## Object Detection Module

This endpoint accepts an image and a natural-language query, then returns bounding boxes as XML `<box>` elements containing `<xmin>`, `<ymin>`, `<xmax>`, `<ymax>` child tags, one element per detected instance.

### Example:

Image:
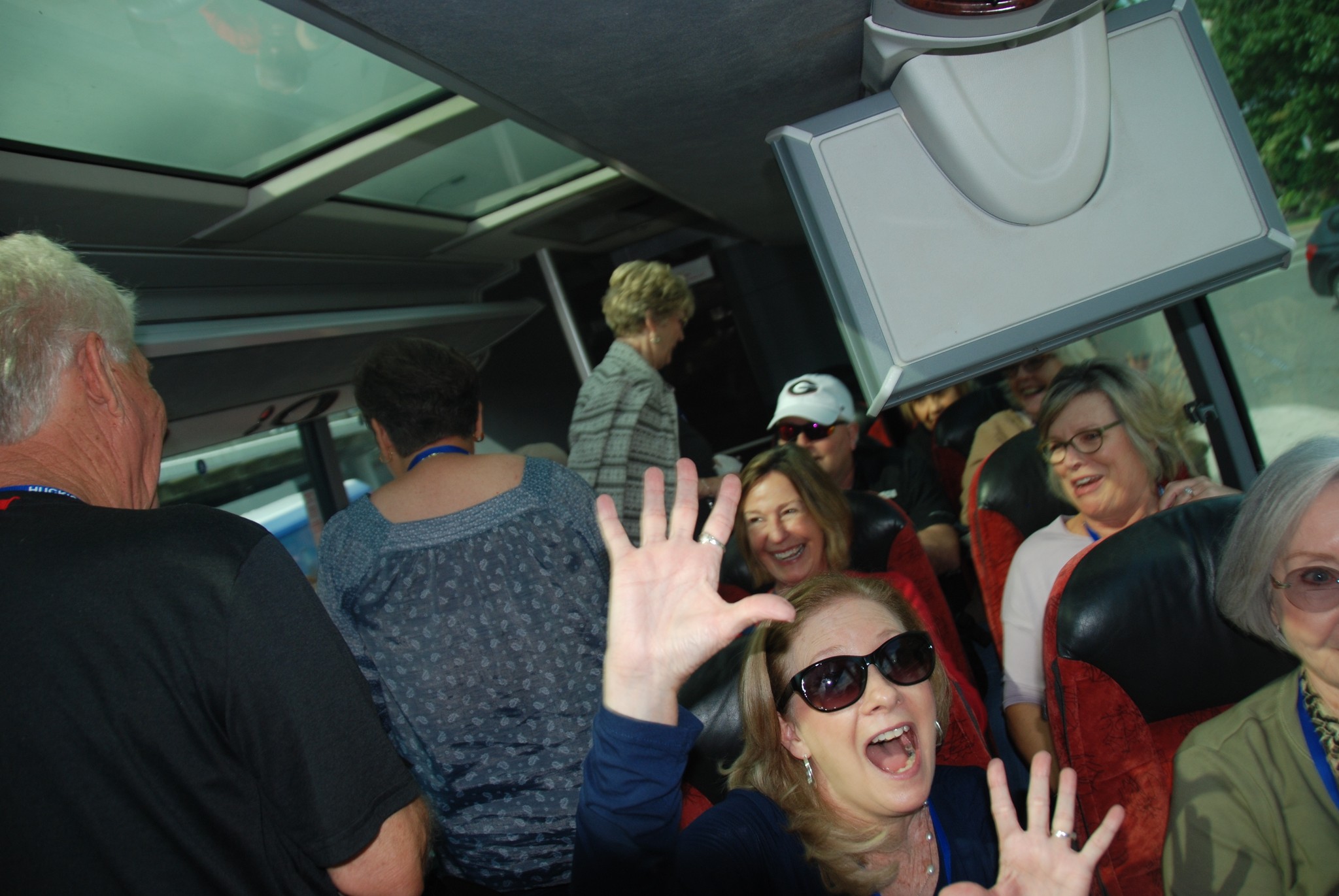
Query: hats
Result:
<box><xmin>767</xmin><ymin>373</ymin><xmax>856</xmax><ymax>430</ymax></box>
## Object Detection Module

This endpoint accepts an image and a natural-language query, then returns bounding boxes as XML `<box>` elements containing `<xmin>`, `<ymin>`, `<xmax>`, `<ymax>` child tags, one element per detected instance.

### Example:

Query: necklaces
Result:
<box><xmin>917</xmin><ymin>799</ymin><xmax>935</xmax><ymax>896</ymax></box>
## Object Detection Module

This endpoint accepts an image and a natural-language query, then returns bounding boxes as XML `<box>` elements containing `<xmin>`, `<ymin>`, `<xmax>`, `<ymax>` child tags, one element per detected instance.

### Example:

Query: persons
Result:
<box><xmin>311</xmin><ymin>260</ymin><xmax>1339</xmax><ymax>896</ymax></box>
<box><xmin>0</xmin><ymin>228</ymin><xmax>434</xmax><ymax>895</ymax></box>
<box><xmin>572</xmin><ymin>453</ymin><xmax>1125</xmax><ymax>896</ymax></box>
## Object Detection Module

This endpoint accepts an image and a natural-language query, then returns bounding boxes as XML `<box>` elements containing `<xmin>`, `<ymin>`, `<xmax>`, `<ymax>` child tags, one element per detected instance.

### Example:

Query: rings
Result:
<box><xmin>1183</xmin><ymin>486</ymin><xmax>1195</xmax><ymax>498</ymax></box>
<box><xmin>697</xmin><ymin>529</ymin><xmax>729</xmax><ymax>555</ymax></box>
<box><xmin>1051</xmin><ymin>830</ymin><xmax>1077</xmax><ymax>840</ymax></box>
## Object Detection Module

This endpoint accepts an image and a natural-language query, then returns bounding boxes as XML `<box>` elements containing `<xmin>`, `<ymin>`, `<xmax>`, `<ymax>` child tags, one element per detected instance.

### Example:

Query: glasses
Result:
<box><xmin>1269</xmin><ymin>565</ymin><xmax>1339</xmax><ymax>611</ymax></box>
<box><xmin>780</xmin><ymin>629</ymin><xmax>935</xmax><ymax>716</ymax></box>
<box><xmin>774</xmin><ymin>420</ymin><xmax>848</xmax><ymax>440</ymax></box>
<box><xmin>1042</xmin><ymin>418</ymin><xmax>1122</xmax><ymax>464</ymax></box>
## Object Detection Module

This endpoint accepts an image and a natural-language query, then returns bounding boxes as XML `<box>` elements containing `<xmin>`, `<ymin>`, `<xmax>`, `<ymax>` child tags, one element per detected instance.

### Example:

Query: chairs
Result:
<box><xmin>679</xmin><ymin>385</ymin><xmax>1301</xmax><ymax>896</ymax></box>
